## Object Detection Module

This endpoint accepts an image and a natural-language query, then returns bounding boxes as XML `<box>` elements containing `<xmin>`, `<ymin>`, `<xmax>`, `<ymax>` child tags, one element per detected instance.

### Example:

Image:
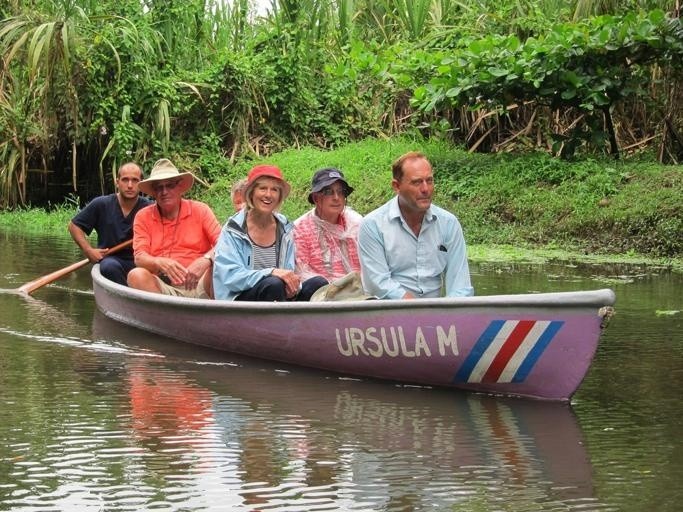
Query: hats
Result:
<box><xmin>308</xmin><ymin>167</ymin><xmax>354</xmax><ymax>205</ymax></box>
<box><xmin>244</xmin><ymin>165</ymin><xmax>291</xmax><ymax>210</ymax></box>
<box><xmin>137</xmin><ymin>158</ymin><xmax>194</xmax><ymax>198</ymax></box>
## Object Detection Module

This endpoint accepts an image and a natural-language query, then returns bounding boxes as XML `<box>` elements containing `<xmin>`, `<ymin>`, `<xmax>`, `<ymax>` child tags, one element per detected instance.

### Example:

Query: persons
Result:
<box><xmin>356</xmin><ymin>153</ymin><xmax>475</xmax><ymax>300</ymax></box>
<box><xmin>292</xmin><ymin>168</ymin><xmax>364</xmax><ymax>282</ymax></box>
<box><xmin>125</xmin><ymin>159</ymin><xmax>222</xmax><ymax>299</ymax></box>
<box><xmin>230</xmin><ymin>178</ymin><xmax>250</xmax><ymax>213</ymax></box>
<box><xmin>211</xmin><ymin>164</ymin><xmax>326</xmax><ymax>301</ymax></box>
<box><xmin>68</xmin><ymin>162</ymin><xmax>152</xmax><ymax>287</ymax></box>
<box><xmin>131</xmin><ymin>348</ymin><xmax>209</xmax><ymax>472</ymax></box>
<box><xmin>292</xmin><ymin>419</ymin><xmax>345</xmax><ymax>488</ymax></box>
<box><xmin>213</xmin><ymin>394</ymin><xmax>300</xmax><ymax>509</ymax></box>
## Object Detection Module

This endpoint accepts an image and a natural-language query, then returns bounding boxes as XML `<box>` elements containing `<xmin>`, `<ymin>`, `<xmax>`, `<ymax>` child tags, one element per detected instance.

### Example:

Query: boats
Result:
<box><xmin>90</xmin><ymin>263</ymin><xmax>615</xmax><ymax>403</ymax></box>
<box><xmin>81</xmin><ymin>308</ymin><xmax>599</xmax><ymax>490</ymax></box>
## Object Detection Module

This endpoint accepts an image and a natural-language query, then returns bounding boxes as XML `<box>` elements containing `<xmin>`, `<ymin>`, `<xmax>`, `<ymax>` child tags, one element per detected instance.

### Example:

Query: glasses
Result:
<box><xmin>320</xmin><ymin>186</ymin><xmax>350</xmax><ymax>198</ymax></box>
<box><xmin>152</xmin><ymin>181</ymin><xmax>176</xmax><ymax>191</ymax></box>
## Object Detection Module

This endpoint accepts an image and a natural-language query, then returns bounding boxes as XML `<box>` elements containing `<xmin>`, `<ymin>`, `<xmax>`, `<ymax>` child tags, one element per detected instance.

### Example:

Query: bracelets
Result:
<box><xmin>203</xmin><ymin>252</ymin><xmax>211</xmax><ymax>260</ymax></box>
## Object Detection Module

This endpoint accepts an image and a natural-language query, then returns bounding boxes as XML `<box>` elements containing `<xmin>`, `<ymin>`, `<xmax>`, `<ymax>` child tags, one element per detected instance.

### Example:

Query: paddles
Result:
<box><xmin>19</xmin><ymin>238</ymin><xmax>136</xmax><ymax>299</ymax></box>
